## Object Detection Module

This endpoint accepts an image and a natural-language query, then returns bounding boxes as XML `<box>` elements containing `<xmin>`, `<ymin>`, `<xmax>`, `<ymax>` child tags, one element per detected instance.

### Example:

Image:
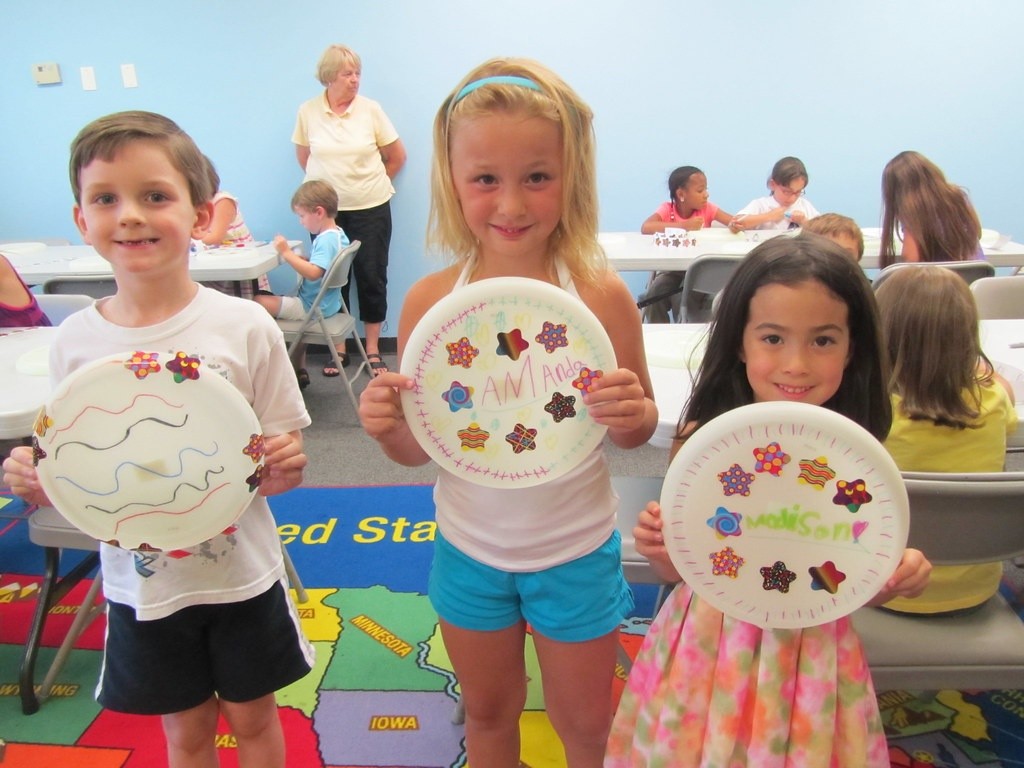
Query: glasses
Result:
<box><xmin>778</xmin><ymin>184</ymin><xmax>805</xmax><ymax>198</ymax></box>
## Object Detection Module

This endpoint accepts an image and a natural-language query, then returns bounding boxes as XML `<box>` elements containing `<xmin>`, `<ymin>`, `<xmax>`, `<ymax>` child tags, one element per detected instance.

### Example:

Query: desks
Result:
<box><xmin>0</xmin><ymin>326</ymin><xmax>103</xmax><ymax>715</ymax></box>
<box><xmin>631</xmin><ymin>318</ymin><xmax>1023</xmax><ymax>467</ymax></box>
<box><xmin>1</xmin><ymin>240</ymin><xmax>302</xmax><ymax>303</ymax></box>
<box><xmin>597</xmin><ymin>228</ymin><xmax>1023</xmax><ymax>313</ymax></box>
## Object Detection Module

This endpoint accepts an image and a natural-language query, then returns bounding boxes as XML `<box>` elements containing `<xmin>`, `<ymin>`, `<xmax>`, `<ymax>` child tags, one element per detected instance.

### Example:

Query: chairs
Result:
<box><xmin>850</xmin><ymin>470</ymin><xmax>1023</xmax><ymax>692</ymax></box>
<box><xmin>678</xmin><ymin>252</ymin><xmax>748</xmax><ymax>322</ymax></box>
<box><xmin>868</xmin><ymin>261</ymin><xmax>995</xmax><ymax>287</ymax></box>
<box><xmin>267</xmin><ymin>239</ymin><xmax>374</xmax><ymax>419</ymax></box>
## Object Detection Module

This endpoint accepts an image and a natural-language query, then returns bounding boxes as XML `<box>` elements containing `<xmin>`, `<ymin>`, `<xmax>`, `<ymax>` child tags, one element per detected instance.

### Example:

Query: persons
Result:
<box><xmin>1</xmin><ymin>111</ymin><xmax>312</xmax><ymax>768</ymax></box>
<box><xmin>868</xmin><ymin>262</ymin><xmax>1019</xmax><ymax>621</ymax></box>
<box><xmin>644</xmin><ymin>151</ymin><xmax>986</xmax><ymax>324</ymax></box>
<box><xmin>0</xmin><ymin>256</ymin><xmax>52</xmax><ymax>328</ymax></box>
<box><xmin>192</xmin><ymin>152</ymin><xmax>273</xmax><ymax>300</ymax></box>
<box><xmin>293</xmin><ymin>45</ymin><xmax>407</xmax><ymax>375</ymax></box>
<box><xmin>255</xmin><ymin>180</ymin><xmax>351</xmax><ymax>390</ymax></box>
<box><xmin>358</xmin><ymin>59</ymin><xmax>658</xmax><ymax>768</ymax></box>
<box><xmin>603</xmin><ymin>235</ymin><xmax>932</xmax><ymax>768</ymax></box>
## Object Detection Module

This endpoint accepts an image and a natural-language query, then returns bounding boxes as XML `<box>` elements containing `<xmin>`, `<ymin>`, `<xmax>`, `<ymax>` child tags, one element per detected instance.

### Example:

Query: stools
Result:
<box><xmin>29</xmin><ymin>469</ymin><xmax>305</xmax><ymax>701</ymax></box>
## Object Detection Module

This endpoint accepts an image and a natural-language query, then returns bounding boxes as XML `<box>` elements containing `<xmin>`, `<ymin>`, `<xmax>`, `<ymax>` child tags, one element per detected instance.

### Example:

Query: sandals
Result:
<box><xmin>295</xmin><ymin>368</ymin><xmax>310</xmax><ymax>389</ymax></box>
<box><xmin>322</xmin><ymin>353</ymin><xmax>350</xmax><ymax>376</ymax></box>
<box><xmin>364</xmin><ymin>354</ymin><xmax>388</xmax><ymax>378</ymax></box>
